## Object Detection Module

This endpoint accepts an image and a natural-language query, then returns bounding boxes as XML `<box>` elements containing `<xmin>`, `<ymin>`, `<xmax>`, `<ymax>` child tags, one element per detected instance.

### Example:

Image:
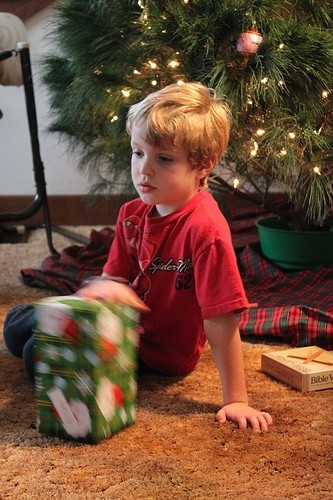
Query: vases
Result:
<box><xmin>255</xmin><ymin>216</ymin><xmax>333</xmax><ymax>269</ymax></box>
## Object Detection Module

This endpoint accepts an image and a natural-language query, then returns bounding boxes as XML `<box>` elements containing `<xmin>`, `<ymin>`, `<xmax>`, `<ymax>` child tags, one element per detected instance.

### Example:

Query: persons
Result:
<box><xmin>3</xmin><ymin>81</ymin><xmax>274</xmax><ymax>433</ymax></box>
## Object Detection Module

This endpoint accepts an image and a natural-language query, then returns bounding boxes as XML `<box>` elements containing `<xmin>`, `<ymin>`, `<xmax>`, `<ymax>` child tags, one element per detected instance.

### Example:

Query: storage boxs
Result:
<box><xmin>30</xmin><ymin>295</ymin><xmax>140</xmax><ymax>444</ymax></box>
<box><xmin>261</xmin><ymin>345</ymin><xmax>332</xmax><ymax>392</ymax></box>
